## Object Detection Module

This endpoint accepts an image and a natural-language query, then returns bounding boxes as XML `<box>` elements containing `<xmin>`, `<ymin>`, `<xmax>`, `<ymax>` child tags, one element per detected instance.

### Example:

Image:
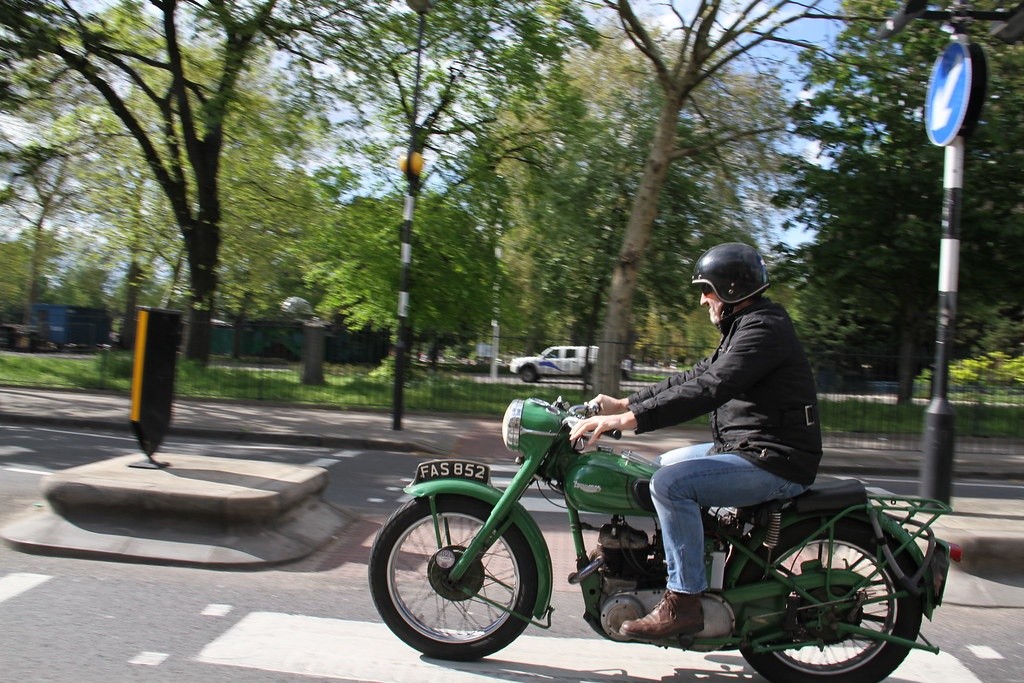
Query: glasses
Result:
<box><xmin>701</xmin><ymin>283</ymin><xmax>714</xmax><ymax>296</ymax></box>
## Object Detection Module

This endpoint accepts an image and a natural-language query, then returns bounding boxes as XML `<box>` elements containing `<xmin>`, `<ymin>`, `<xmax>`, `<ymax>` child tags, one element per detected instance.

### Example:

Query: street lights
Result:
<box><xmin>388</xmin><ymin>147</ymin><xmax>426</xmax><ymax>430</ymax></box>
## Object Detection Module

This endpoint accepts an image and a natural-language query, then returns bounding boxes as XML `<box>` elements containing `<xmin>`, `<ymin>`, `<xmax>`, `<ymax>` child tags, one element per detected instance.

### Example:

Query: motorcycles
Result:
<box><xmin>367</xmin><ymin>396</ymin><xmax>964</xmax><ymax>683</ymax></box>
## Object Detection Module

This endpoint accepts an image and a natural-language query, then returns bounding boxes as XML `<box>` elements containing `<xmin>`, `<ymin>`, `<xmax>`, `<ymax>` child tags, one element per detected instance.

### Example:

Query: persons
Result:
<box><xmin>569</xmin><ymin>243</ymin><xmax>823</xmax><ymax>640</ymax></box>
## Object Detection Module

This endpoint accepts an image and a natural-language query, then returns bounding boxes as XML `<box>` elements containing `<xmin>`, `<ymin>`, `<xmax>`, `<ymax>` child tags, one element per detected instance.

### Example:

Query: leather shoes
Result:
<box><xmin>618</xmin><ymin>589</ymin><xmax>704</xmax><ymax>640</ymax></box>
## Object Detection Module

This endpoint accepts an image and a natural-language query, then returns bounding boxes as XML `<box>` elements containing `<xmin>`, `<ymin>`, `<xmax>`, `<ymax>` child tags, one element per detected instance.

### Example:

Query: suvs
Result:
<box><xmin>509</xmin><ymin>345</ymin><xmax>597</xmax><ymax>382</ymax></box>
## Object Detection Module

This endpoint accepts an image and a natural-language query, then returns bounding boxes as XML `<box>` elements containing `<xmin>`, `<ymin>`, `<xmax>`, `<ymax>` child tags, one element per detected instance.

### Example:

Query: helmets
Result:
<box><xmin>691</xmin><ymin>242</ymin><xmax>770</xmax><ymax>304</ymax></box>
